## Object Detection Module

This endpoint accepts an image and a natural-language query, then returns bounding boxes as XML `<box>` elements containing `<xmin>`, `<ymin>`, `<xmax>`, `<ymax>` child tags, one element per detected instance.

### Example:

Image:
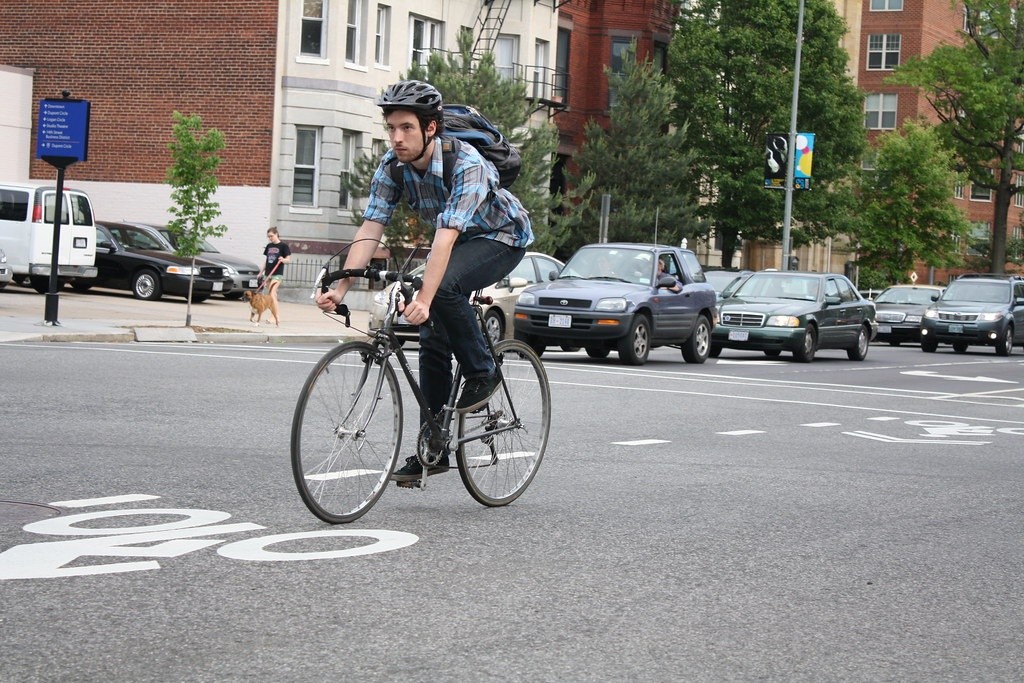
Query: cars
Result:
<box><xmin>709</xmin><ymin>271</ymin><xmax>878</xmax><ymax>364</ymax></box>
<box><xmin>712</xmin><ymin>271</ymin><xmax>818</xmax><ymax>328</ymax></box>
<box><xmin>67</xmin><ymin>221</ymin><xmax>234</xmax><ymax>304</ymax></box>
<box><xmin>119</xmin><ymin>222</ymin><xmax>264</xmax><ymax>300</ymax></box>
<box><xmin>0</xmin><ymin>243</ymin><xmax>13</xmax><ymax>289</ymax></box>
<box><xmin>871</xmin><ymin>284</ymin><xmax>946</xmax><ymax>345</ymax></box>
<box><xmin>512</xmin><ymin>241</ymin><xmax>718</xmax><ymax>365</ymax></box>
<box><xmin>370</xmin><ymin>252</ymin><xmax>582</xmax><ymax>351</ymax></box>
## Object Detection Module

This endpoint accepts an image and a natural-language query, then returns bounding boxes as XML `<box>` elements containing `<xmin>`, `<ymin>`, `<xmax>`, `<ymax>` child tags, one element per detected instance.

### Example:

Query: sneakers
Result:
<box><xmin>456</xmin><ymin>367</ymin><xmax>503</xmax><ymax>413</ymax></box>
<box><xmin>390</xmin><ymin>454</ymin><xmax>449</xmax><ymax>481</ymax></box>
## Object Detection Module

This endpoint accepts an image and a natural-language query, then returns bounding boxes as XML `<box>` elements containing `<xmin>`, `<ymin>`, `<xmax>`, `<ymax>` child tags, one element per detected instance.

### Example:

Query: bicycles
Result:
<box><xmin>290</xmin><ymin>236</ymin><xmax>552</xmax><ymax>525</ymax></box>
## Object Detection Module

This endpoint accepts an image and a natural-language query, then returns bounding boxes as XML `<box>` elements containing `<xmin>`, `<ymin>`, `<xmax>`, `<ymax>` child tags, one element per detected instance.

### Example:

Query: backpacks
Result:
<box><xmin>390</xmin><ymin>104</ymin><xmax>522</xmax><ymax>194</ymax></box>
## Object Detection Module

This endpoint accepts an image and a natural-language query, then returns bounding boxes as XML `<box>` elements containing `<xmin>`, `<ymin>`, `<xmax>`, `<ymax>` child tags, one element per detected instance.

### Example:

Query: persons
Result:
<box><xmin>657</xmin><ymin>259</ymin><xmax>682</xmax><ymax>292</ymax></box>
<box><xmin>258</xmin><ymin>226</ymin><xmax>291</xmax><ymax>323</ymax></box>
<box><xmin>589</xmin><ymin>253</ymin><xmax>615</xmax><ymax>277</ymax></box>
<box><xmin>315</xmin><ymin>79</ymin><xmax>536</xmax><ymax>482</ymax></box>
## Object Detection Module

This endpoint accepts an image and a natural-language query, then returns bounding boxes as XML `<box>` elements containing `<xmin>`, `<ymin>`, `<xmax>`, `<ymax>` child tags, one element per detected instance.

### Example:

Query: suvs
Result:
<box><xmin>921</xmin><ymin>271</ymin><xmax>1024</xmax><ymax>357</ymax></box>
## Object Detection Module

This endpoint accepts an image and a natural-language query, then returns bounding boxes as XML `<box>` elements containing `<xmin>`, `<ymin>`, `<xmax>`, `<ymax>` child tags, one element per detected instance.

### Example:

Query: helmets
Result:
<box><xmin>377</xmin><ymin>80</ymin><xmax>442</xmax><ymax>115</ymax></box>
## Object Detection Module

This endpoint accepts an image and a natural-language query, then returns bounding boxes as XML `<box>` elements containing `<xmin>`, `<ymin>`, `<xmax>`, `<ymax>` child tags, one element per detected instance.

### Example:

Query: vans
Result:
<box><xmin>0</xmin><ymin>182</ymin><xmax>99</xmax><ymax>293</ymax></box>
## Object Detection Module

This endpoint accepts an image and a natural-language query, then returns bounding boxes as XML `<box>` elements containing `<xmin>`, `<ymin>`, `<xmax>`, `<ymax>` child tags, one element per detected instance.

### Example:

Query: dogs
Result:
<box><xmin>241</xmin><ymin>282</ymin><xmax>280</xmax><ymax>326</ymax></box>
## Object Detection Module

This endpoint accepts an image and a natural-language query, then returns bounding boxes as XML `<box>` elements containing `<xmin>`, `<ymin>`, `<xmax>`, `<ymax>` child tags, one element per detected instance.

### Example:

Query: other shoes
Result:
<box><xmin>275</xmin><ymin>320</ymin><xmax>279</xmax><ymax>329</ymax></box>
<box><xmin>265</xmin><ymin>319</ymin><xmax>274</xmax><ymax>324</ymax></box>
